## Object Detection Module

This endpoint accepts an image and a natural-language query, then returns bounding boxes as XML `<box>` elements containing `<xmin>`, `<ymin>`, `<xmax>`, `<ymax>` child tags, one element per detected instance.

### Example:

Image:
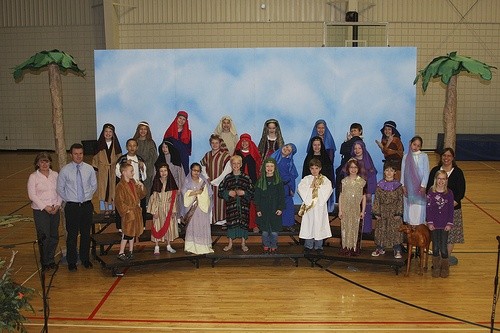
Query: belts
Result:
<box><xmin>66</xmin><ymin>200</ymin><xmax>91</xmax><ymax>207</ymax></box>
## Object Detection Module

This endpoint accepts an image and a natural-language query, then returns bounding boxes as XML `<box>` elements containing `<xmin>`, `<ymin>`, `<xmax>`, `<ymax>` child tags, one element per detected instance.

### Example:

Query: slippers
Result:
<box><xmin>223</xmin><ymin>246</ymin><xmax>232</xmax><ymax>251</ymax></box>
<box><xmin>241</xmin><ymin>244</ymin><xmax>249</xmax><ymax>251</ymax></box>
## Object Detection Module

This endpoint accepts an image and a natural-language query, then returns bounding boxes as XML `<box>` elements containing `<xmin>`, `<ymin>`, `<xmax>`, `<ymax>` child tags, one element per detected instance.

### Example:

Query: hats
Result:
<box><xmin>380</xmin><ymin>121</ymin><xmax>401</xmax><ymax>137</ymax></box>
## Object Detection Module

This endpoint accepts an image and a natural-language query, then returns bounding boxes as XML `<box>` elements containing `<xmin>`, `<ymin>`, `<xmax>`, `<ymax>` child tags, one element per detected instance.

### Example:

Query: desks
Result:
<box><xmin>438</xmin><ymin>133</ymin><xmax>500</xmax><ymax>161</ymax></box>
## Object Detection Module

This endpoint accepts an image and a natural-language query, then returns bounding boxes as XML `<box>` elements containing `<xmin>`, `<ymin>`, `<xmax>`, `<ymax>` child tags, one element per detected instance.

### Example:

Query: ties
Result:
<box><xmin>76</xmin><ymin>164</ymin><xmax>85</xmax><ymax>203</ymax></box>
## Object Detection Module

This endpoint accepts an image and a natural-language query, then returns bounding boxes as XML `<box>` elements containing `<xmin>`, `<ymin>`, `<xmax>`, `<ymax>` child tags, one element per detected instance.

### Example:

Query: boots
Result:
<box><xmin>431</xmin><ymin>255</ymin><xmax>441</xmax><ymax>277</ymax></box>
<box><xmin>440</xmin><ymin>258</ymin><xmax>450</xmax><ymax>278</ymax></box>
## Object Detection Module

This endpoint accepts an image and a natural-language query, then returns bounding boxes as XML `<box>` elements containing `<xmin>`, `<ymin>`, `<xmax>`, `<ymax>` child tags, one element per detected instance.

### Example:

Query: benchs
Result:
<box><xmin>88</xmin><ymin>212</ymin><xmax>417</xmax><ymax>277</ymax></box>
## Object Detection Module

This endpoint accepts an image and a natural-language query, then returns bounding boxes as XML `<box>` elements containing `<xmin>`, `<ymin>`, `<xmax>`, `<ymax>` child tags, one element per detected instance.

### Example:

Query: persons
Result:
<box><xmin>374</xmin><ymin>121</ymin><xmax>404</xmax><ymax>182</ymax></box>
<box><xmin>302</xmin><ymin>119</ymin><xmax>337</xmax><ymax>216</ymax></box>
<box><xmin>154</xmin><ymin>139</ymin><xmax>186</xmax><ymax>225</ymax></box>
<box><xmin>426</xmin><ymin>147</ymin><xmax>467</xmax><ymax>265</ymax></box>
<box><xmin>399</xmin><ymin>135</ymin><xmax>430</xmax><ymax>226</ymax></box>
<box><xmin>181</xmin><ymin>116</ymin><xmax>299</xmax><ymax>255</ymax></box>
<box><xmin>90</xmin><ymin>123</ymin><xmax>122</xmax><ymax>219</ymax></box>
<box><xmin>340</xmin><ymin>140</ymin><xmax>377</xmax><ymax>239</ymax></box>
<box><xmin>337</xmin><ymin>157</ymin><xmax>368</xmax><ymax>258</ymax></box>
<box><xmin>164</xmin><ymin>111</ymin><xmax>192</xmax><ymax>177</ymax></box>
<box><xmin>57</xmin><ymin>143</ymin><xmax>97</xmax><ymax>272</ymax></box>
<box><xmin>426</xmin><ymin>170</ymin><xmax>454</xmax><ymax>278</ymax></box>
<box><xmin>114</xmin><ymin>139</ymin><xmax>148</xmax><ymax>190</ymax></box>
<box><xmin>27</xmin><ymin>153</ymin><xmax>63</xmax><ymax>273</ymax></box>
<box><xmin>340</xmin><ymin>123</ymin><xmax>366</xmax><ymax>163</ymax></box>
<box><xmin>146</xmin><ymin>162</ymin><xmax>179</xmax><ymax>256</ymax></box>
<box><xmin>371</xmin><ymin>163</ymin><xmax>404</xmax><ymax>259</ymax></box>
<box><xmin>114</xmin><ymin>163</ymin><xmax>148</xmax><ymax>262</ymax></box>
<box><xmin>297</xmin><ymin>159</ymin><xmax>333</xmax><ymax>262</ymax></box>
<box><xmin>131</xmin><ymin>121</ymin><xmax>157</xmax><ymax>214</ymax></box>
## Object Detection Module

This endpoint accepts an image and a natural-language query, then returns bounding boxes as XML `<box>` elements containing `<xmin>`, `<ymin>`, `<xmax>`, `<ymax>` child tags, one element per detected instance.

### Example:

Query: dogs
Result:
<box><xmin>397</xmin><ymin>223</ymin><xmax>430</xmax><ymax>278</ymax></box>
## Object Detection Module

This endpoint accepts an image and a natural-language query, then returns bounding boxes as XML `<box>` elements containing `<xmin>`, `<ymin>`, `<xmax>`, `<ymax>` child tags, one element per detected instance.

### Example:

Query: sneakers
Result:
<box><xmin>394</xmin><ymin>248</ymin><xmax>403</xmax><ymax>259</ymax></box>
<box><xmin>372</xmin><ymin>248</ymin><xmax>385</xmax><ymax>257</ymax></box>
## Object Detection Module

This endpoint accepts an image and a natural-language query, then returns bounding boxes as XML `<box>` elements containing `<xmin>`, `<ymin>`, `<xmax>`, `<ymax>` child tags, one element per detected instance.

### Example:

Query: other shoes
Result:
<box><xmin>41</xmin><ymin>262</ymin><xmax>58</xmax><ymax>272</ymax></box>
<box><xmin>304</xmin><ymin>247</ymin><xmax>324</xmax><ymax>255</ymax></box>
<box><xmin>286</xmin><ymin>225</ymin><xmax>296</xmax><ymax>232</ymax></box>
<box><xmin>264</xmin><ymin>246</ymin><xmax>279</xmax><ymax>253</ymax></box>
<box><xmin>68</xmin><ymin>262</ymin><xmax>77</xmax><ymax>271</ymax></box>
<box><xmin>337</xmin><ymin>247</ymin><xmax>360</xmax><ymax>256</ymax></box>
<box><xmin>323</xmin><ymin>242</ymin><xmax>330</xmax><ymax>247</ymax></box>
<box><xmin>81</xmin><ymin>259</ymin><xmax>94</xmax><ymax>269</ymax></box>
<box><xmin>222</xmin><ymin>224</ymin><xmax>227</xmax><ymax>230</ymax></box>
<box><xmin>127</xmin><ymin>251</ymin><xmax>134</xmax><ymax>261</ymax></box>
<box><xmin>253</xmin><ymin>227</ymin><xmax>259</xmax><ymax>233</ymax></box>
<box><xmin>119</xmin><ymin>253</ymin><xmax>129</xmax><ymax>261</ymax></box>
<box><xmin>404</xmin><ymin>246</ymin><xmax>433</xmax><ymax>254</ymax></box>
<box><xmin>297</xmin><ymin>239</ymin><xmax>305</xmax><ymax>246</ymax></box>
<box><xmin>167</xmin><ymin>246</ymin><xmax>176</xmax><ymax>253</ymax></box>
<box><xmin>154</xmin><ymin>249</ymin><xmax>160</xmax><ymax>256</ymax></box>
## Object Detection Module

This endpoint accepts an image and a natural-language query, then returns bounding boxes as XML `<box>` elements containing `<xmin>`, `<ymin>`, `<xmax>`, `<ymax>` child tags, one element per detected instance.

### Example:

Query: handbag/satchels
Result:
<box><xmin>180</xmin><ymin>197</ymin><xmax>198</xmax><ymax>226</ymax></box>
